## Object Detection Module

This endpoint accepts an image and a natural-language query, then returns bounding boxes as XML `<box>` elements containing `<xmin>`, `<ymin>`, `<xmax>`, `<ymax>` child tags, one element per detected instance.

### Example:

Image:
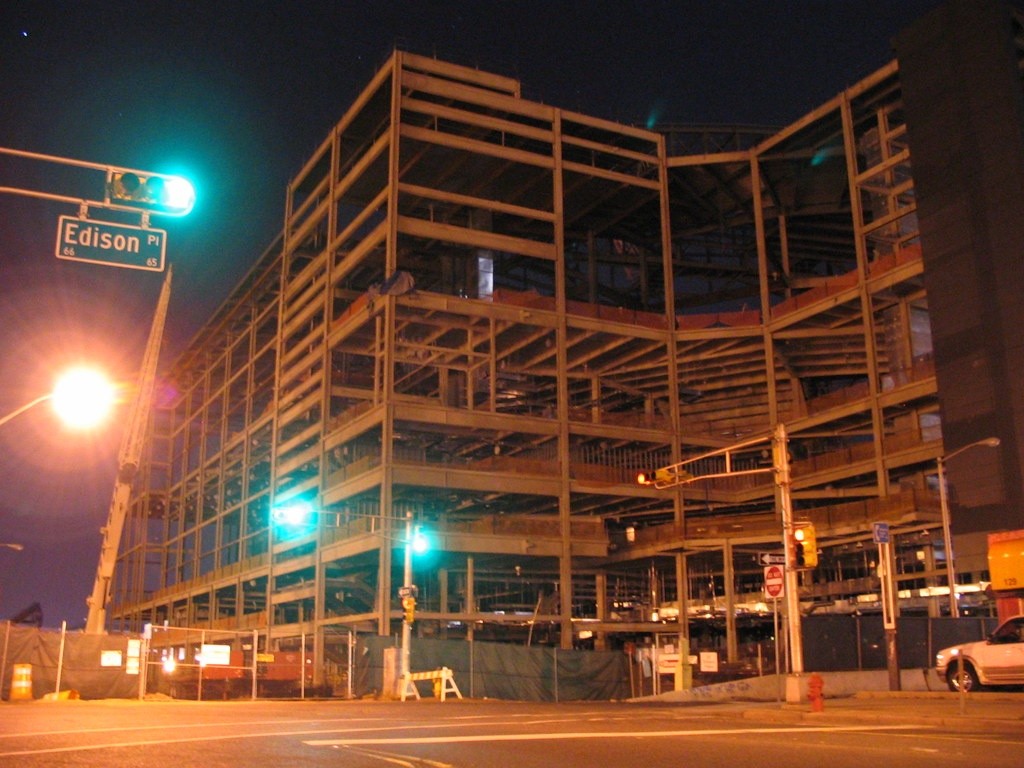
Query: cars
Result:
<box><xmin>935</xmin><ymin>614</ymin><xmax>1024</xmax><ymax>693</ymax></box>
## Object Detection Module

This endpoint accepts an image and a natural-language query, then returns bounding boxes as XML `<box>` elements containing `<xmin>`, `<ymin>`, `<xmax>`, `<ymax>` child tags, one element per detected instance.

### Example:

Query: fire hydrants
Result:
<box><xmin>806</xmin><ymin>672</ymin><xmax>826</xmax><ymax>712</ymax></box>
<box><xmin>433</xmin><ymin>667</ymin><xmax>442</xmax><ymax>699</ymax></box>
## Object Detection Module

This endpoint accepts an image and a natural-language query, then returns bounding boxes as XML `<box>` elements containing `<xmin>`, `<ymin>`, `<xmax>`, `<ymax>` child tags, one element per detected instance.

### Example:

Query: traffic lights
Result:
<box><xmin>773</xmin><ymin>446</ymin><xmax>794</xmax><ymax>486</ymax></box>
<box><xmin>111</xmin><ymin>171</ymin><xmax>192</xmax><ymax>208</ymax></box>
<box><xmin>638</xmin><ymin>468</ymin><xmax>676</xmax><ymax>485</ymax></box>
<box><xmin>794</xmin><ymin>526</ymin><xmax>818</xmax><ymax>568</ymax></box>
<box><xmin>401</xmin><ymin>597</ymin><xmax>414</xmax><ymax>624</ymax></box>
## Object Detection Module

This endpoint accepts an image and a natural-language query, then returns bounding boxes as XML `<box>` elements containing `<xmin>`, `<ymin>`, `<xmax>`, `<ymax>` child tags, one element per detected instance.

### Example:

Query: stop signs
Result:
<box><xmin>763</xmin><ymin>564</ymin><xmax>785</xmax><ymax>599</ymax></box>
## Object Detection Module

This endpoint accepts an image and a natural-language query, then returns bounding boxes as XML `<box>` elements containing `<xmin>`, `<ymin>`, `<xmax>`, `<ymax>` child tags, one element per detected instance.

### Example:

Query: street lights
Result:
<box><xmin>936</xmin><ymin>435</ymin><xmax>1002</xmax><ymax>617</ymax></box>
